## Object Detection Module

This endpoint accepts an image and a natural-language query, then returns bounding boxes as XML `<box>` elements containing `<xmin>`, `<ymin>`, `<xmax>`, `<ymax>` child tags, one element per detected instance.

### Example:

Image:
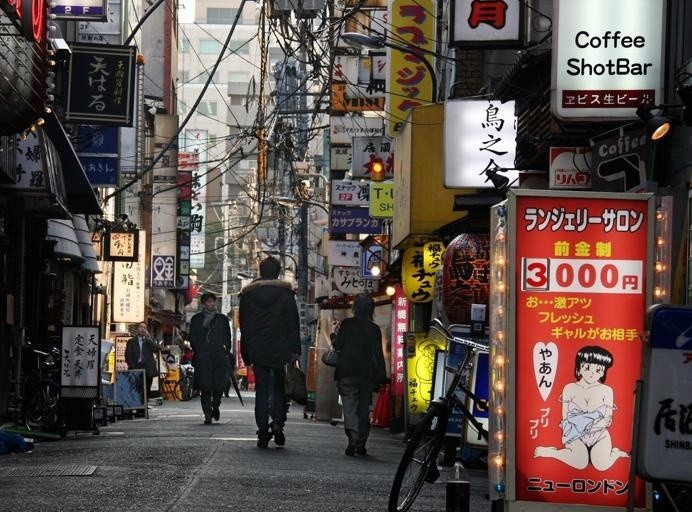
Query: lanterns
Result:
<box><xmin>400</xmin><ymin>230</ymin><xmax>490</xmax><ymax>334</ymax></box>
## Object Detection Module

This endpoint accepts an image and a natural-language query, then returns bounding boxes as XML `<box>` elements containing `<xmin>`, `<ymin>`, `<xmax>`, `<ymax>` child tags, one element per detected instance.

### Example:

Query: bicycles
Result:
<box><xmin>18</xmin><ymin>340</ymin><xmax>62</xmax><ymax>432</ymax></box>
<box><xmin>389</xmin><ymin>319</ymin><xmax>492</xmax><ymax>512</ymax></box>
<box><xmin>173</xmin><ymin>365</ymin><xmax>202</xmax><ymax>401</ymax></box>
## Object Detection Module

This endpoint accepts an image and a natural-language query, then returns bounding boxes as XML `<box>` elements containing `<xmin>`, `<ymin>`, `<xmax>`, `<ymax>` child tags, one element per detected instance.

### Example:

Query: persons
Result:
<box><xmin>125</xmin><ymin>321</ymin><xmax>160</xmax><ymax>418</ymax></box>
<box><xmin>221</xmin><ymin>353</ymin><xmax>236</xmax><ymax>398</ymax></box>
<box><xmin>180</xmin><ymin>347</ymin><xmax>192</xmax><ymax>364</ymax></box>
<box><xmin>188</xmin><ymin>292</ymin><xmax>232</xmax><ymax>425</ymax></box>
<box><xmin>533</xmin><ymin>345</ymin><xmax>629</xmax><ymax>473</ymax></box>
<box><xmin>329</xmin><ymin>292</ymin><xmax>387</xmax><ymax>456</ymax></box>
<box><xmin>237</xmin><ymin>255</ymin><xmax>302</xmax><ymax>449</ymax></box>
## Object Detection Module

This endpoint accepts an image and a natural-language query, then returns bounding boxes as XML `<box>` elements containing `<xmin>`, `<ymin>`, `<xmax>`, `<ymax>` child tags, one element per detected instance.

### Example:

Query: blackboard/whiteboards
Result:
<box><xmin>113</xmin><ymin>368</ymin><xmax>147</xmax><ymax>409</ymax></box>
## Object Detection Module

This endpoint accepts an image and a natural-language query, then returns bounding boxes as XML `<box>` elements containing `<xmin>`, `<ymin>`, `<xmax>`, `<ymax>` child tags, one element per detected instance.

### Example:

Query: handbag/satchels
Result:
<box><xmin>322</xmin><ymin>337</ymin><xmax>339</xmax><ymax>367</ymax></box>
<box><xmin>283</xmin><ymin>355</ymin><xmax>309</xmax><ymax>406</ymax></box>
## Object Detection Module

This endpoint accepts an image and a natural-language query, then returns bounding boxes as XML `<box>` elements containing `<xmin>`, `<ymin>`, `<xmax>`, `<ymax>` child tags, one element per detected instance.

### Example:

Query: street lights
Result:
<box><xmin>278</xmin><ymin>197</ymin><xmax>349</xmax><ymax>336</ymax></box>
<box><xmin>235</xmin><ymin>271</ymin><xmax>257</xmax><ymax>284</ymax></box>
<box><xmin>261</xmin><ymin>249</ymin><xmax>311</xmax><ymax>382</ymax></box>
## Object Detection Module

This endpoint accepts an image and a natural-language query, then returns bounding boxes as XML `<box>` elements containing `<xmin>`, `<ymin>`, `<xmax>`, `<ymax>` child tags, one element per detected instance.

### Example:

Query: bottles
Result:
<box><xmin>446</xmin><ymin>446</ymin><xmax>470</xmax><ymax>512</ymax></box>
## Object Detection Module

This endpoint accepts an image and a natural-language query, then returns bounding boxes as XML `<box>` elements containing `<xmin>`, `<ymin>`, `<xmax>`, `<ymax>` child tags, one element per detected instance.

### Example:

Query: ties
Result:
<box><xmin>141</xmin><ymin>336</ymin><xmax>145</xmax><ymax>363</ymax></box>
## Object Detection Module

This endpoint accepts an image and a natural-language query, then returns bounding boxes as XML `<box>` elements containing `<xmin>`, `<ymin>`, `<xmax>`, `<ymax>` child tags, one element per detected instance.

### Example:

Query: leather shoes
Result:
<box><xmin>204</xmin><ymin>414</ymin><xmax>213</xmax><ymax>425</ymax></box>
<box><xmin>356</xmin><ymin>447</ymin><xmax>368</xmax><ymax>454</ymax></box>
<box><xmin>257</xmin><ymin>438</ymin><xmax>269</xmax><ymax>449</ymax></box>
<box><xmin>273</xmin><ymin>429</ymin><xmax>286</xmax><ymax>446</ymax></box>
<box><xmin>212</xmin><ymin>406</ymin><xmax>220</xmax><ymax>421</ymax></box>
<box><xmin>345</xmin><ymin>444</ymin><xmax>357</xmax><ymax>457</ymax></box>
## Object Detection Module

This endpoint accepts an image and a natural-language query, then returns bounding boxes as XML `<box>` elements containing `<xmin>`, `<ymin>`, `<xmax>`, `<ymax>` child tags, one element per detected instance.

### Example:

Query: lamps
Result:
<box><xmin>634</xmin><ymin>101</ymin><xmax>683</xmax><ymax>141</ymax></box>
<box><xmin>485</xmin><ymin>167</ymin><xmax>525</xmax><ymax>189</ymax></box>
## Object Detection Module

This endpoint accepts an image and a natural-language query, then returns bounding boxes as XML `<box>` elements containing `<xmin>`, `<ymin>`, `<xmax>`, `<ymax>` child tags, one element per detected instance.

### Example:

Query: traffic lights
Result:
<box><xmin>371</xmin><ymin>163</ymin><xmax>385</xmax><ymax>181</ymax></box>
